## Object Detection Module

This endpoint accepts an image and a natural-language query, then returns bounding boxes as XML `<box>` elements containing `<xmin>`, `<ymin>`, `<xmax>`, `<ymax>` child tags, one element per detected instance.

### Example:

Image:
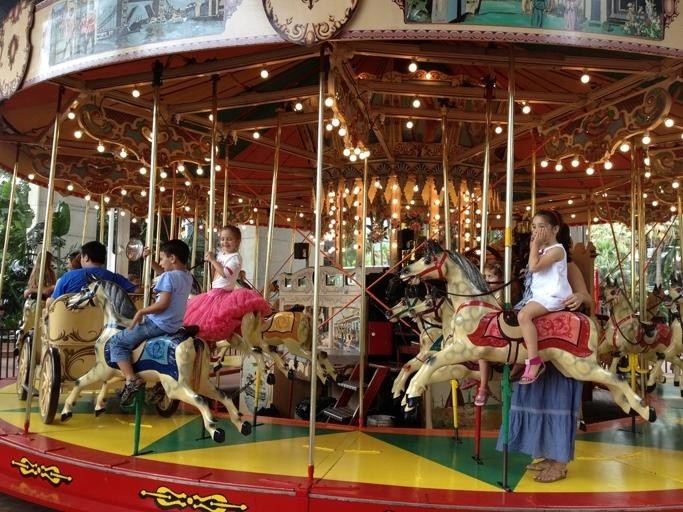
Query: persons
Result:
<box><xmin>183</xmin><ymin>224</ymin><xmax>272</xmax><ymax>342</ymax></box>
<box><xmin>19</xmin><ymin>245</ymin><xmax>140</xmax><ymax>320</ymax></box>
<box><xmin>473</xmin><ymin>209</ymin><xmax>594</xmax><ymax>482</ymax></box>
<box><xmin>109</xmin><ymin>239</ymin><xmax>194</xmax><ymax>410</ymax></box>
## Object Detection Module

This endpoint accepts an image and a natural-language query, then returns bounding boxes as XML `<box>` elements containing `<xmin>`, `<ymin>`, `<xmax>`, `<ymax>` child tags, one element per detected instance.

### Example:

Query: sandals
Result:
<box><xmin>462</xmin><ymin>379</ymin><xmax>477</xmax><ymax>389</ymax></box>
<box><xmin>518</xmin><ymin>356</ymin><xmax>545</xmax><ymax>385</ymax></box>
<box><xmin>474</xmin><ymin>386</ymin><xmax>490</xmax><ymax>407</ymax></box>
<box><xmin>526</xmin><ymin>458</ymin><xmax>554</xmax><ymax>470</ymax></box>
<box><xmin>535</xmin><ymin>463</ymin><xmax>568</xmax><ymax>482</ymax></box>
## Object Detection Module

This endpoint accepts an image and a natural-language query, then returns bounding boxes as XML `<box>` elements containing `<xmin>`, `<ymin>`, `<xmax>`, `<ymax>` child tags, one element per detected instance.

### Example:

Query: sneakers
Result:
<box><xmin>145</xmin><ymin>381</ymin><xmax>164</xmax><ymax>407</ymax></box>
<box><xmin>121</xmin><ymin>376</ymin><xmax>144</xmax><ymax>406</ymax></box>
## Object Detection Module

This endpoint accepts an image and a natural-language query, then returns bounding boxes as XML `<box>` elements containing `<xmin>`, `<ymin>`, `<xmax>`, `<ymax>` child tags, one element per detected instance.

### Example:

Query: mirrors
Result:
<box><xmin>318</xmin><ymin>174</ymin><xmax>504</xmax><ymax>272</ymax></box>
